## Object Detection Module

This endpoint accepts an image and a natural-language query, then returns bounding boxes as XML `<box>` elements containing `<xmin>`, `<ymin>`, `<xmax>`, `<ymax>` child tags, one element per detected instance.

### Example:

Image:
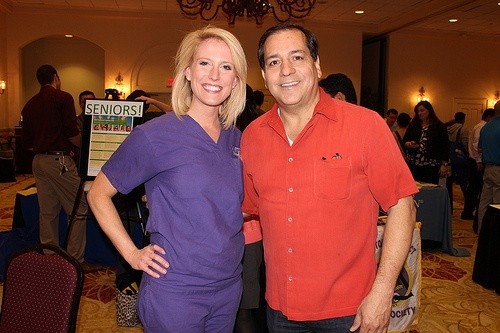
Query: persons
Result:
<box><xmin>318</xmin><ymin>73</ymin><xmax>358</xmax><ymax>105</ymax></box>
<box><xmin>447</xmin><ymin>112</ymin><xmax>470</xmax><ymax>215</ymax></box>
<box><xmin>478</xmin><ymin>100</ymin><xmax>500</xmax><ymax>235</ymax></box>
<box><xmin>233</xmin><ymin>83</ymin><xmax>266</xmax><ymax>333</ymax></box>
<box><xmin>72</xmin><ymin>90</ymin><xmax>173</xmax><ymax>327</ymax></box>
<box><xmin>461</xmin><ymin>108</ymin><xmax>495</xmax><ymax>235</ymax></box>
<box><xmin>402</xmin><ymin>101</ymin><xmax>450</xmax><ymax>185</ymax></box>
<box><xmin>142</xmin><ymin>24</ymin><xmax>419</xmax><ymax>333</ymax></box>
<box><xmin>390</xmin><ymin>113</ymin><xmax>411</xmax><ymax>159</ymax></box>
<box><xmin>88</xmin><ymin>27</ymin><xmax>247</xmax><ymax>333</ymax></box>
<box><xmin>21</xmin><ymin>65</ymin><xmax>103</xmax><ymax>273</ymax></box>
<box><xmin>383</xmin><ymin>109</ymin><xmax>398</xmax><ymax>131</ymax></box>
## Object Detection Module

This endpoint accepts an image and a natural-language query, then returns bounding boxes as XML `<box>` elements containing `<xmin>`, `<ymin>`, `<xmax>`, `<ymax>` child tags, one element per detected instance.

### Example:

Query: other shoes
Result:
<box><xmin>461</xmin><ymin>211</ymin><xmax>474</xmax><ymax>219</ymax></box>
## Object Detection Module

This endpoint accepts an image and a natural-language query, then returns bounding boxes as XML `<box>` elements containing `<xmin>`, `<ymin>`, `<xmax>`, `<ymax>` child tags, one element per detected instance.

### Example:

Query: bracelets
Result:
<box><xmin>441</xmin><ymin>163</ymin><xmax>448</xmax><ymax>166</ymax></box>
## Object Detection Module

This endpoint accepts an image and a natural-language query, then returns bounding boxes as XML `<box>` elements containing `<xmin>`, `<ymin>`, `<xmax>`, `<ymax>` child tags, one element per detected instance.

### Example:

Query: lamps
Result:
<box><xmin>418</xmin><ymin>86</ymin><xmax>425</xmax><ymax>96</ymax></box>
<box><xmin>493</xmin><ymin>91</ymin><xmax>500</xmax><ymax>100</ymax></box>
<box><xmin>115</xmin><ymin>72</ymin><xmax>123</xmax><ymax>85</ymax></box>
<box><xmin>177</xmin><ymin>0</ymin><xmax>316</xmax><ymax>27</ymax></box>
<box><xmin>0</xmin><ymin>81</ymin><xmax>6</xmax><ymax>94</ymax></box>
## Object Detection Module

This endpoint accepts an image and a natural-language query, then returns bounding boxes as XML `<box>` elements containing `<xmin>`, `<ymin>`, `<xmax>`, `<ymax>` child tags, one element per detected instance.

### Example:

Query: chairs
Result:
<box><xmin>0</xmin><ymin>242</ymin><xmax>84</xmax><ymax>333</ymax></box>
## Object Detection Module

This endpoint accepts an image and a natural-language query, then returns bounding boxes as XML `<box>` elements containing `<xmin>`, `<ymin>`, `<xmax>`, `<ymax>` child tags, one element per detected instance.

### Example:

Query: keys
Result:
<box><xmin>59</xmin><ymin>165</ymin><xmax>68</xmax><ymax>176</ymax></box>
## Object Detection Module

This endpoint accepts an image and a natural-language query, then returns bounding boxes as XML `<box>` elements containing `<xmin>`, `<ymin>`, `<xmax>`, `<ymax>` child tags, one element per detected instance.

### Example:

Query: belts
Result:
<box><xmin>37</xmin><ymin>151</ymin><xmax>70</xmax><ymax>155</ymax></box>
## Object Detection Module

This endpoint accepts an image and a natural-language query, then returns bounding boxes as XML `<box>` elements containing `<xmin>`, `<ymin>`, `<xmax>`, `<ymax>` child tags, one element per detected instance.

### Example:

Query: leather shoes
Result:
<box><xmin>79</xmin><ymin>260</ymin><xmax>102</xmax><ymax>272</ymax></box>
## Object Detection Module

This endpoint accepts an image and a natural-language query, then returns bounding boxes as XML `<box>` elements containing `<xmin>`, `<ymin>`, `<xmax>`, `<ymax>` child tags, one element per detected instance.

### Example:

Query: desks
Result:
<box><xmin>471</xmin><ymin>203</ymin><xmax>500</xmax><ymax>296</ymax></box>
<box><xmin>412</xmin><ymin>181</ymin><xmax>452</xmax><ymax>259</ymax></box>
<box><xmin>375</xmin><ymin>210</ymin><xmax>422</xmax><ymax>333</ymax></box>
